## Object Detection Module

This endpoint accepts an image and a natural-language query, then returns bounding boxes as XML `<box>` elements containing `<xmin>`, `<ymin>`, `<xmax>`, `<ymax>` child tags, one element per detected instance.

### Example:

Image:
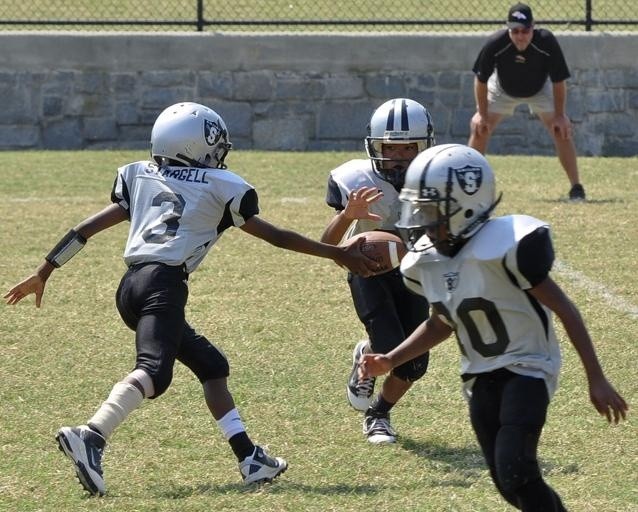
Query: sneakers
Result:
<box><xmin>568</xmin><ymin>184</ymin><xmax>587</xmax><ymax>203</ymax></box>
<box><xmin>346</xmin><ymin>338</ymin><xmax>378</xmax><ymax>412</ymax></box>
<box><xmin>363</xmin><ymin>405</ymin><xmax>398</xmax><ymax>445</ymax></box>
<box><xmin>236</xmin><ymin>445</ymin><xmax>289</xmax><ymax>486</ymax></box>
<box><xmin>55</xmin><ymin>423</ymin><xmax>107</xmax><ymax>497</ymax></box>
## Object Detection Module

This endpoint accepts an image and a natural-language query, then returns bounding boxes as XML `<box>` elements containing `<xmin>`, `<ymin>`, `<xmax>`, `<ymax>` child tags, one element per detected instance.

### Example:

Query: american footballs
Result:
<box><xmin>334</xmin><ymin>231</ymin><xmax>406</xmax><ymax>276</ymax></box>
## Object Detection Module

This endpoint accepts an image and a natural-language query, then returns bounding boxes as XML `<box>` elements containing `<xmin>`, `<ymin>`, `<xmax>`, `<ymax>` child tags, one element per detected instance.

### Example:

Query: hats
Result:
<box><xmin>507</xmin><ymin>1</ymin><xmax>533</xmax><ymax>29</ymax></box>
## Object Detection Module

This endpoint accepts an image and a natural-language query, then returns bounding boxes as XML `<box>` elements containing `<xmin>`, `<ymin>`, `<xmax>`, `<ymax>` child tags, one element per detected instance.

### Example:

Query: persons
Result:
<box><xmin>320</xmin><ymin>98</ymin><xmax>435</xmax><ymax>445</ymax></box>
<box><xmin>4</xmin><ymin>102</ymin><xmax>381</xmax><ymax>496</ymax></box>
<box><xmin>467</xmin><ymin>1</ymin><xmax>586</xmax><ymax>201</ymax></box>
<box><xmin>358</xmin><ymin>144</ymin><xmax>630</xmax><ymax>512</ymax></box>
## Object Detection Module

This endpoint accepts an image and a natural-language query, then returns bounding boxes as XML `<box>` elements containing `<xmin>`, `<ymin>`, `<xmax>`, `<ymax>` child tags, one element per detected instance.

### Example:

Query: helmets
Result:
<box><xmin>149</xmin><ymin>102</ymin><xmax>233</xmax><ymax>171</ymax></box>
<box><xmin>363</xmin><ymin>98</ymin><xmax>436</xmax><ymax>184</ymax></box>
<box><xmin>394</xmin><ymin>143</ymin><xmax>499</xmax><ymax>254</ymax></box>
